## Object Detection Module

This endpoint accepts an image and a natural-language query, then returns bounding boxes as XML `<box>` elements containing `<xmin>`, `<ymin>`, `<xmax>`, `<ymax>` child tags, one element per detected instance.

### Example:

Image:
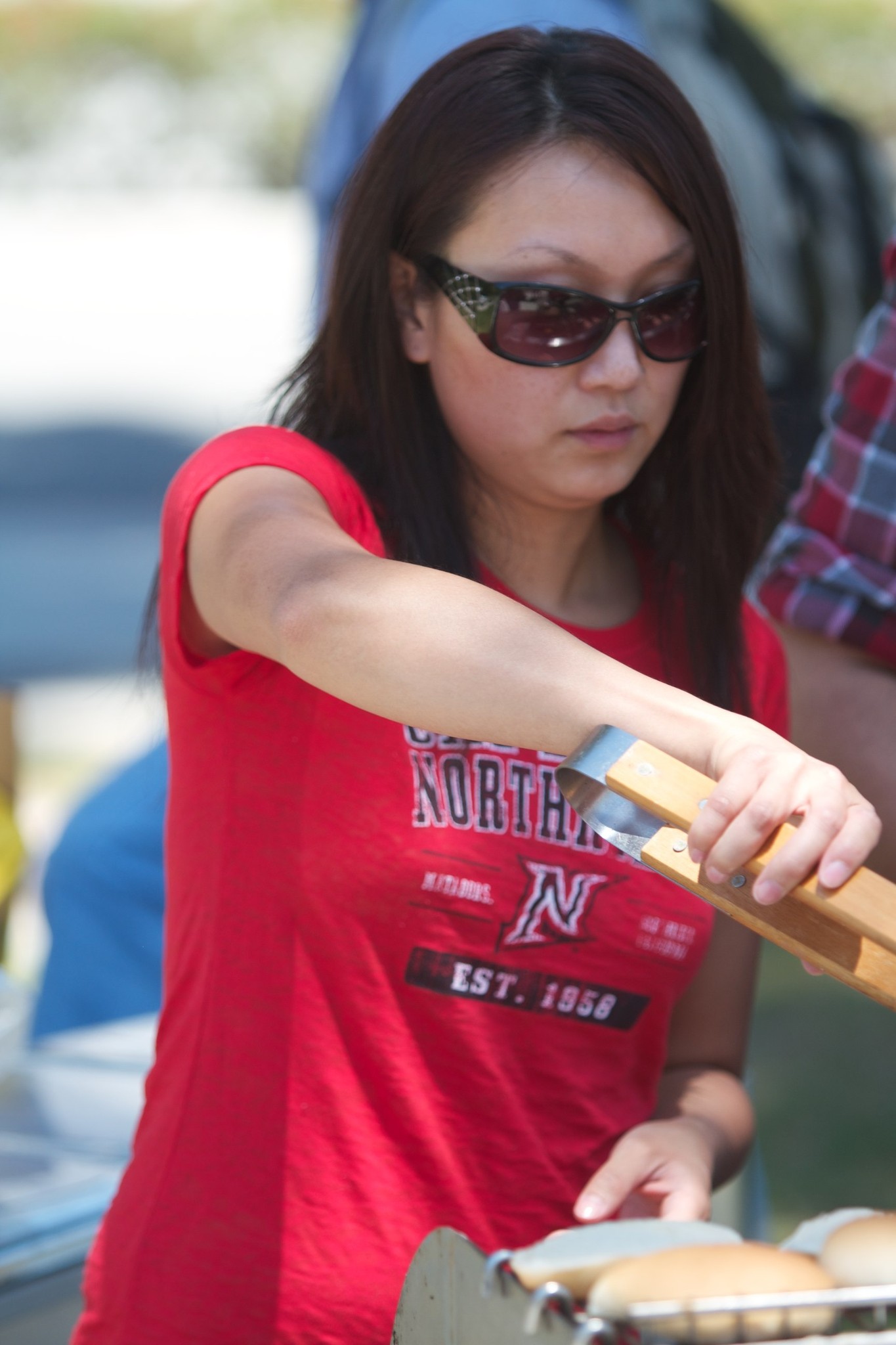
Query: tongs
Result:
<box><xmin>553</xmin><ymin>722</ymin><xmax>896</xmax><ymax>1009</ymax></box>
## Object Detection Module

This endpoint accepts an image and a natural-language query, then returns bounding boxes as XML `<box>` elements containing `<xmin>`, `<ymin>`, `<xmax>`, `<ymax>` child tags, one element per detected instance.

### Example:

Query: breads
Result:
<box><xmin>505</xmin><ymin>1209</ymin><xmax>896</xmax><ymax>1341</ymax></box>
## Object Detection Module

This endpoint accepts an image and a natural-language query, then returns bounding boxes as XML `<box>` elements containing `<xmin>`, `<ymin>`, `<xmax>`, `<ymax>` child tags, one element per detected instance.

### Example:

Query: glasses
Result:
<box><xmin>394</xmin><ymin>249</ymin><xmax>706</xmax><ymax>369</ymax></box>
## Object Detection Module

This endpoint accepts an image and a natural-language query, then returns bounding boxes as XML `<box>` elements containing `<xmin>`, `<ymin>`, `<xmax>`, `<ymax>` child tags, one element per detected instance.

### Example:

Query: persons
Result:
<box><xmin>73</xmin><ymin>24</ymin><xmax>881</xmax><ymax>1345</ymax></box>
<box><xmin>315</xmin><ymin>0</ymin><xmax>896</xmax><ymax>978</ymax></box>
